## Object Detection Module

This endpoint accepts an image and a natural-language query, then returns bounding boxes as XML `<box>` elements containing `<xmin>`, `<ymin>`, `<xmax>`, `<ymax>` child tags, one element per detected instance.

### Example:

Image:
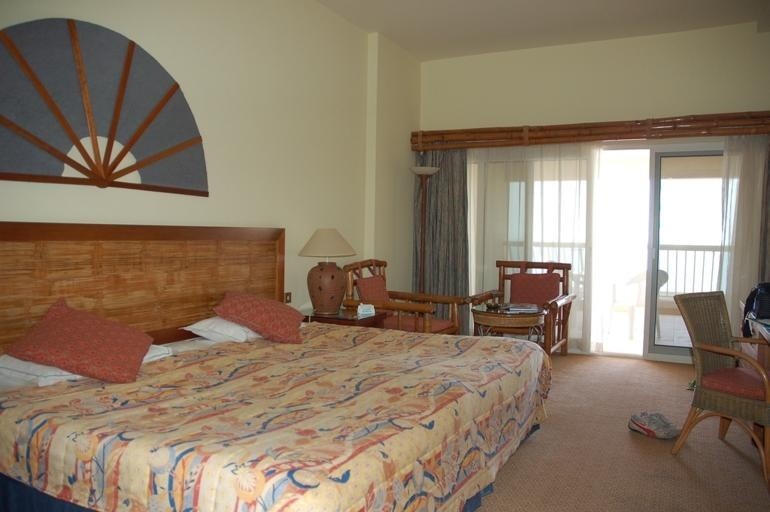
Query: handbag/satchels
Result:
<box><xmin>743</xmin><ymin>282</ymin><xmax>770</xmax><ymax>337</ymax></box>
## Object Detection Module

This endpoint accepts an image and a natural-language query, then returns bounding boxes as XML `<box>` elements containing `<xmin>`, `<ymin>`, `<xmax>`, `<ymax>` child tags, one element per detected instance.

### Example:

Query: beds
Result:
<box><xmin>0</xmin><ymin>222</ymin><xmax>545</xmax><ymax>512</ymax></box>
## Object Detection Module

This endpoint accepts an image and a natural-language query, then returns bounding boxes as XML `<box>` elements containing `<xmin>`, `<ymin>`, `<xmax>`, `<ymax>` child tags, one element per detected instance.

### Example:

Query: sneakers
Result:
<box><xmin>629</xmin><ymin>411</ymin><xmax>682</xmax><ymax>439</ymax></box>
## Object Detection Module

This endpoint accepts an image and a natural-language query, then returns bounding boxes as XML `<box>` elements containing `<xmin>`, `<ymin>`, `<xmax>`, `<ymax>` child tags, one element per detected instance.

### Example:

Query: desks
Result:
<box><xmin>738</xmin><ymin>298</ymin><xmax>769</xmax><ymax>450</ymax></box>
<box><xmin>470</xmin><ymin>303</ymin><xmax>547</xmax><ymax>345</ymax></box>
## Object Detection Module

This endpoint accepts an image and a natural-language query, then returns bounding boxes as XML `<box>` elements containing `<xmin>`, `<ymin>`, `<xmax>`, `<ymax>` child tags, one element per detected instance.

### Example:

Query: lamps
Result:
<box><xmin>411</xmin><ymin>165</ymin><xmax>440</xmax><ymax>319</ymax></box>
<box><xmin>299</xmin><ymin>227</ymin><xmax>357</xmax><ymax>316</ymax></box>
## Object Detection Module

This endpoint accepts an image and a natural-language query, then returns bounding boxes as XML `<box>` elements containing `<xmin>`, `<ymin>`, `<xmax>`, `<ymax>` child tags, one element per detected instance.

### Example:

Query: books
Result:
<box><xmin>506</xmin><ymin>304</ymin><xmax>539</xmax><ymax>313</ymax></box>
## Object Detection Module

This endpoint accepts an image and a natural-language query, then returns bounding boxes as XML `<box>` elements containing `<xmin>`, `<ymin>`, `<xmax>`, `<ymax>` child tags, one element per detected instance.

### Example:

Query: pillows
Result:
<box><xmin>215</xmin><ymin>293</ymin><xmax>305</xmax><ymax>346</ymax></box>
<box><xmin>355</xmin><ymin>275</ymin><xmax>394</xmax><ymax>317</ymax></box>
<box><xmin>179</xmin><ymin>315</ymin><xmax>262</xmax><ymax>345</ymax></box>
<box><xmin>7</xmin><ymin>295</ymin><xmax>156</xmax><ymax>382</ymax></box>
<box><xmin>510</xmin><ymin>273</ymin><xmax>560</xmax><ymax>305</ymax></box>
<box><xmin>0</xmin><ymin>344</ymin><xmax>173</xmax><ymax>388</ymax></box>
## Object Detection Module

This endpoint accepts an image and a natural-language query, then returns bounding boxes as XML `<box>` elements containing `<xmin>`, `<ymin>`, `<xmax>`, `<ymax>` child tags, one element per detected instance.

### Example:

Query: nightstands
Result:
<box><xmin>300</xmin><ymin>307</ymin><xmax>388</xmax><ymax>328</ymax></box>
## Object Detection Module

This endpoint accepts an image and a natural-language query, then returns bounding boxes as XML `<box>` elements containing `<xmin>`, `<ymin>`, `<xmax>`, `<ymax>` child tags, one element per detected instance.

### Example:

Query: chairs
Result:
<box><xmin>670</xmin><ymin>291</ymin><xmax>770</xmax><ymax>484</ymax></box>
<box><xmin>466</xmin><ymin>259</ymin><xmax>577</xmax><ymax>368</ymax></box>
<box><xmin>343</xmin><ymin>259</ymin><xmax>465</xmax><ymax>335</ymax></box>
